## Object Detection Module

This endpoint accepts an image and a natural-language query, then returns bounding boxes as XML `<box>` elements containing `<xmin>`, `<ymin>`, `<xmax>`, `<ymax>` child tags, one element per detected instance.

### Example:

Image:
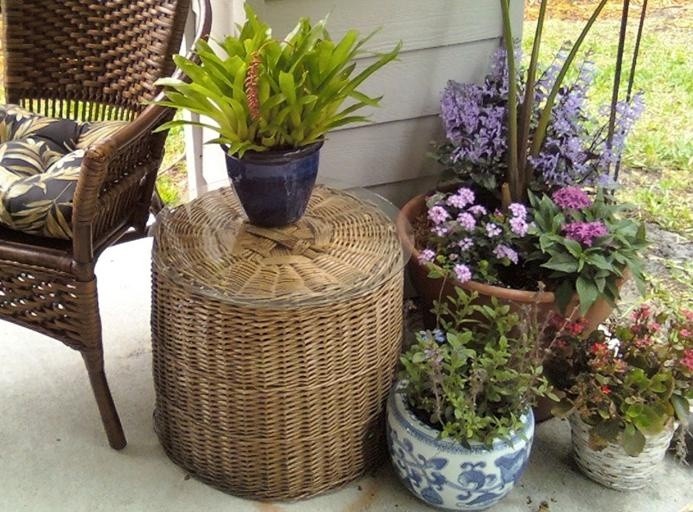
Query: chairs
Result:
<box><xmin>0</xmin><ymin>1</ymin><xmax>212</xmax><ymax>451</ymax></box>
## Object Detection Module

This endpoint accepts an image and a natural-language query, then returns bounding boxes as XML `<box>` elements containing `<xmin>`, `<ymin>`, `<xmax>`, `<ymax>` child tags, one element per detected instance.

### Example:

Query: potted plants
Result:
<box><xmin>137</xmin><ymin>2</ymin><xmax>403</xmax><ymax>229</ymax></box>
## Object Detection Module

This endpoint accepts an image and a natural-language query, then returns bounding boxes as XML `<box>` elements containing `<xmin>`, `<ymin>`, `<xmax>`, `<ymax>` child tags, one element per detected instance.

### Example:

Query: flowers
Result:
<box><xmin>400</xmin><ymin>37</ymin><xmax>692</xmax><ymax>456</ymax></box>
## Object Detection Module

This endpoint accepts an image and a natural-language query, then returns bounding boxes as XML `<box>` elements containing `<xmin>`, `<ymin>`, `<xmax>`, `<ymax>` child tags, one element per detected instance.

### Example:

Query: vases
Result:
<box><xmin>386</xmin><ymin>182</ymin><xmax>680</xmax><ymax>511</ymax></box>
